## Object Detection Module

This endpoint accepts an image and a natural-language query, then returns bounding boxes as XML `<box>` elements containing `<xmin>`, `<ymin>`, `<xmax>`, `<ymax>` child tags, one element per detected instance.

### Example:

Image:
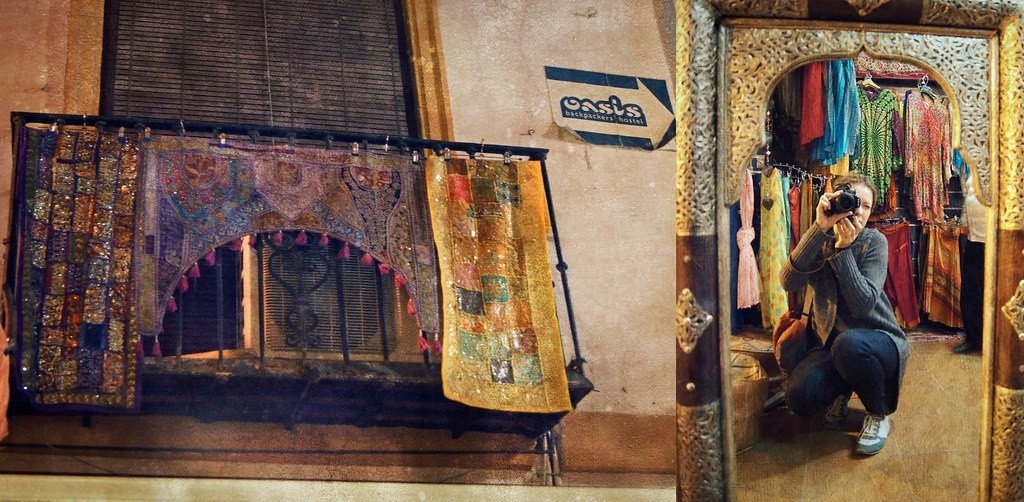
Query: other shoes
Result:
<box><xmin>955</xmin><ymin>342</ymin><xmax>976</xmax><ymax>352</ymax></box>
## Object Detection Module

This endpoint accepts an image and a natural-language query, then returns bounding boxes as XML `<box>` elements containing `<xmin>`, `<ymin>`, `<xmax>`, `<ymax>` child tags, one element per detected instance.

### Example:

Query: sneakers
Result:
<box><xmin>825</xmin><ymin>393</ymin><xmax>852</xmax><ymax>429</ymax></box>
<box><xmin>855</xmin><ymin>413</ymin><xmax>890</xmax><ymax>455</ymax></box>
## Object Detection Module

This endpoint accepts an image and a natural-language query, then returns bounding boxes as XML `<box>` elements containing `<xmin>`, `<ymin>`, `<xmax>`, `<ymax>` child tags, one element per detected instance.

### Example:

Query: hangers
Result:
<box><xmin>759</xmin><ymin>162</ymin><xmax>964</xmax><ymax>228</ymax></box>
<box><xmin>856</xmin><ymin>73</ymin><xmax>937</xmax><ymax>101</ymax></box>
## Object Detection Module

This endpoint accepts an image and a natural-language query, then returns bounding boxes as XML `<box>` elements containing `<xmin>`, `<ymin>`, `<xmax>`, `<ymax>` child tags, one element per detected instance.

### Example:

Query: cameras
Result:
<box><xmin>825</xmin><ymin>187</ymin><xmax>861</xmax><ymax>221</ymax></box>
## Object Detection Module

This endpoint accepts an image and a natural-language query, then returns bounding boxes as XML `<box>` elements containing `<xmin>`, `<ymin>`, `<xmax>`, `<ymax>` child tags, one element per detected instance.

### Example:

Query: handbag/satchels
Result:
<box><xmin>772</xmin><ymin>309</ymin><xmax>816</xmax><ymax>381</ymax></box>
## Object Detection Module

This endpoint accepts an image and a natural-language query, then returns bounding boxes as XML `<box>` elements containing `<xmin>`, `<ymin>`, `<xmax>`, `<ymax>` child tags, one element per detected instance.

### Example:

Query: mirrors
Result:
<box><xmin>666</xmin><ymin>0</ymin><xmax>1021</xmax><ymax>502</ymax></box>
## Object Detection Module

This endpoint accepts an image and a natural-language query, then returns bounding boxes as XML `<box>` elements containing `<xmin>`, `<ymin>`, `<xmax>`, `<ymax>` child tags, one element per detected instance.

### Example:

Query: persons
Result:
<box><xmin>951</xmin><ymin>178</ymin><xmax>987</xmax><ymax>355</ymax></box>
<box><xmin>781</xmin><ymin>174</ymin><xmax>912</xmax><ymax>455</ymax></box>
